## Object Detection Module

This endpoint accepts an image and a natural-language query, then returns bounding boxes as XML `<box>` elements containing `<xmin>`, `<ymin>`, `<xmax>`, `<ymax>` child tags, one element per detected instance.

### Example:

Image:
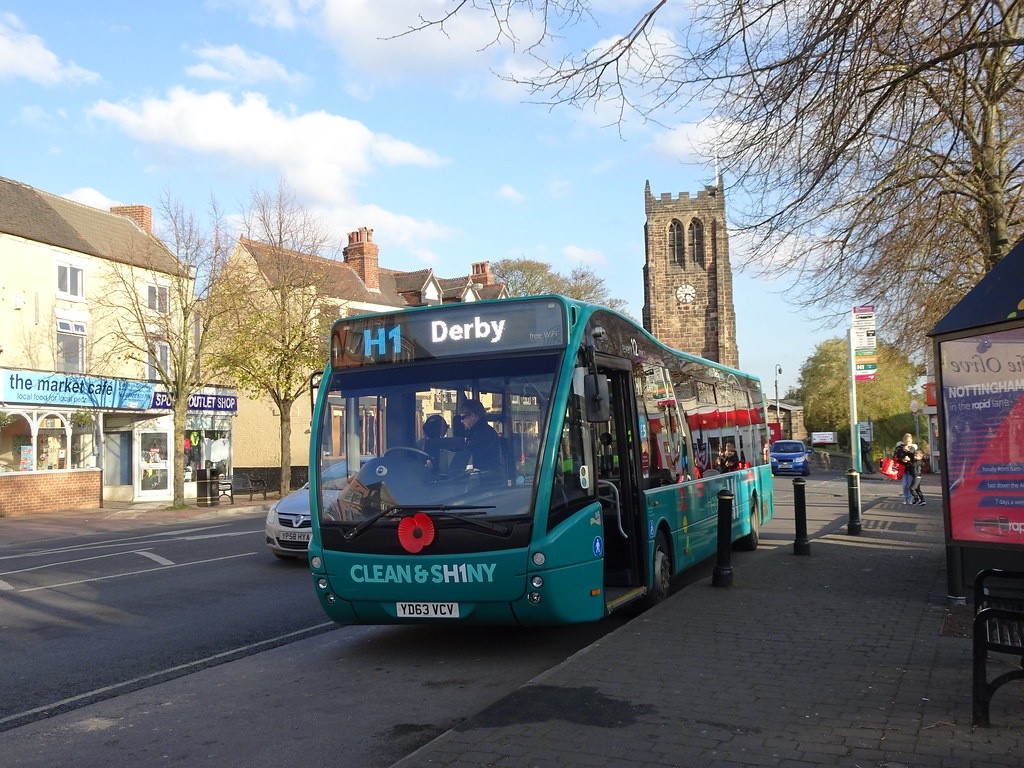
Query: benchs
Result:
<box><xmin>972</xmin><ymin>569</ymin><xmax>1024</xmax><ymax>727</ymax></box>
<box><xmin>218</xmin><ymin>474</ymin><xmax>267</xmax><ymax>505</ymax></box>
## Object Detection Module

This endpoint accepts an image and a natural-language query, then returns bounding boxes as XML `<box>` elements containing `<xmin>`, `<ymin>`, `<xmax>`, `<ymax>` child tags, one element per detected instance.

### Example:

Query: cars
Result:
<box><xmin>264</xmin><ymin>456</ymin><xmax>377</xmax><ymax>561</ymax></box>
<box><xmin>771</xmin><ymin>439</ymin><xmax>812</xmax><ymax>477</ymax></box>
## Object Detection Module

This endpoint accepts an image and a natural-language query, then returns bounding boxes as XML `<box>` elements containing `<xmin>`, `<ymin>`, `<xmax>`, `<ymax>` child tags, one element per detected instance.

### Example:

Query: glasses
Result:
<box><xmin>458</xmin><ymin>414</ymin><xmax>467</xmax><ymax>420</ymax></box>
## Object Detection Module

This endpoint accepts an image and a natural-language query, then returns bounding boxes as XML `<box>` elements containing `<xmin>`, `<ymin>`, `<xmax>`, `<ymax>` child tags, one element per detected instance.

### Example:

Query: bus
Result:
<box><xmin>307</xmin><ymin>294</ymin><xmax>775</xmax><ymax>629</ymax></box>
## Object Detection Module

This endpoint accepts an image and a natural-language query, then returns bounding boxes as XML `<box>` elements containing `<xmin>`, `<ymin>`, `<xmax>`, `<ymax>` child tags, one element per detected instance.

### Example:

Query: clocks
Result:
<box><xmin>676</xmin><ymin>283</ymin><xmax>697</xmax><ymax>304</ymax></box>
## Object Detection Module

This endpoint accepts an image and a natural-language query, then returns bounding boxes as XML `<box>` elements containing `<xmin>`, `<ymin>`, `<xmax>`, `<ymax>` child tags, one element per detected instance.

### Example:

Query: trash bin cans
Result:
<box><xmin>196</xmin><ymin>468</ymin><xmax>220</xmax><ymax>507</ymax></box>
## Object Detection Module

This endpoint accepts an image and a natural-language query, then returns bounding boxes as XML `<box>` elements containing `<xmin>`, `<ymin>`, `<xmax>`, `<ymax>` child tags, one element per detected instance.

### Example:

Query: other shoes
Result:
<box><xmin>913</xmin><ymin>499</ymin><xmax>921</xmax><ymax>505</ymax></box>
<box><xmin>902</xmin><ymin>501</ymin><xmax>907</xmax><ymax>505</ymax></box>
<box><xmin>918</xmin><ymin>500</ymin><xmax>927</xmax><ymax>506</ymax></box>
<box><xmin>865</xmin><ymin>471</ymin><xmax>872</xmax><ymax>474</ymax></box>
<box><xmin>908</xmin><ymin>501</ymin><xmax>912</xmax><ymax>504</ymax></box>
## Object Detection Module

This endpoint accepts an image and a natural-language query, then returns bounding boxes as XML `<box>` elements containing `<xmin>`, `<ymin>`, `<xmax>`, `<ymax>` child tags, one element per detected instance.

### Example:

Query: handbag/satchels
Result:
<box><xmin>879</xmin><ymin>458</ymin><xmax>906</xmax><ymax>482</ymax></box>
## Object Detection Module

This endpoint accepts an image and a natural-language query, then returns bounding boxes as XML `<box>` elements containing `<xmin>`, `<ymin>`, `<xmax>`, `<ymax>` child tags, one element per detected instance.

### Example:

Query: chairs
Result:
<box><xmin>792</xmin><ymin>446</ymin><xmax>800</xmax><ymax>451</ymax></box>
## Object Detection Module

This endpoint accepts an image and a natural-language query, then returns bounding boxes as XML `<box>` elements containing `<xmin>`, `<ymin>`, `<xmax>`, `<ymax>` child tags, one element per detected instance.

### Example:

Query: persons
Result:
<box><xmin>861</xmin><ymin>436</ymin><xmax>875</xmax><ymax>474</ymax></box>
<box><xmin>695</xmin><ymin>442</ymin><xmax>742</xmax><ymax>480</ymax></box>
<box><xmin>425</xmin><ymin>400</ymin><xmax>502</xmax><ymax>487</ymax></box>
<box><xmin>893</xmin><ymin>433</ymin><xmax>927</xmax><ymax>506</ymax></box>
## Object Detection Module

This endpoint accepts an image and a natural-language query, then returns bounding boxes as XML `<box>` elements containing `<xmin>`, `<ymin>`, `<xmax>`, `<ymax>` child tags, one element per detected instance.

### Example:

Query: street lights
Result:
<box><xmin>910</xmin><ymin>399</ymin><xmax>921</xmax><ymax>450</ymax></box>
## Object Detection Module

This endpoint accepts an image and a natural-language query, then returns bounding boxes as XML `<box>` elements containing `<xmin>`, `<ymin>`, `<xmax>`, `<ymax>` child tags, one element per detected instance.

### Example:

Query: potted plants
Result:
<box><xmin>70</xmin><ymin>410</ymin><xmax>95</xmax><ymax>432</ymax></box>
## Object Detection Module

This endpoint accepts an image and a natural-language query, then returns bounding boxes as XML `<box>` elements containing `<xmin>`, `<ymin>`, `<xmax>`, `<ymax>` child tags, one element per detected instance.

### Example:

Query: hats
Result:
<box><xmin>459</xmin><ymin>400</ymin><xmax>486</xmax><ymax>416</ymax></box>
<box><xmin>422</xmin><ymin>414</ymin><xmax>450</xmax><ymax>437</ymax></box>
